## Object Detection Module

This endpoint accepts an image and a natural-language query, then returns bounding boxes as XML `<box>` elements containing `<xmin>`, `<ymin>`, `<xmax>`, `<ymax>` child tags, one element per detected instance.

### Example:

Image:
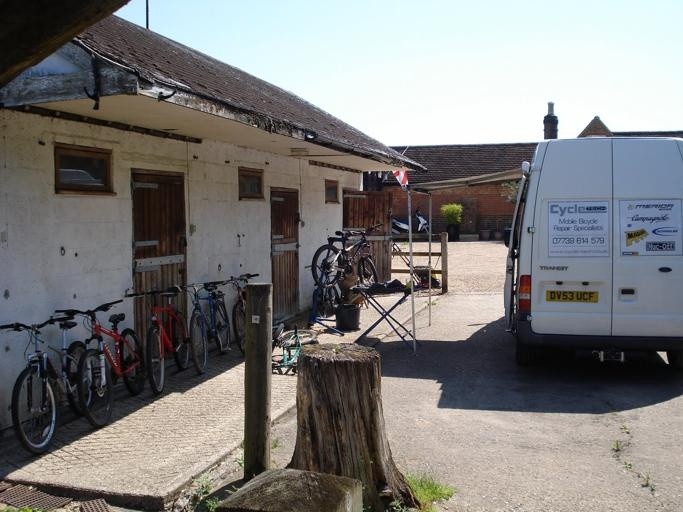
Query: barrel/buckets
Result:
<box><xmin>333</xmin><ymin>302</ymin><xmax>360</xmax><ymax>331</ymax></box>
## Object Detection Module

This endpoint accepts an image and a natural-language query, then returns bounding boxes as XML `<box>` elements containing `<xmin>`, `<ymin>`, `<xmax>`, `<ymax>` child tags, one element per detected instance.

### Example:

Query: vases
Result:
<box><xmin>480</xmin><ymin>230</ymin><xmax>503</xmax><ymax>240</ymax></box>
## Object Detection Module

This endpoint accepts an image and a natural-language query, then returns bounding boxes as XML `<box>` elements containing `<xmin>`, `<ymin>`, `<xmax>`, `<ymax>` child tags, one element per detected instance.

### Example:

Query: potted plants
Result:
<box><xmin>441</xmin><ymin>202</ymin><xmax>463</xmax><ymax>241</ymax></box>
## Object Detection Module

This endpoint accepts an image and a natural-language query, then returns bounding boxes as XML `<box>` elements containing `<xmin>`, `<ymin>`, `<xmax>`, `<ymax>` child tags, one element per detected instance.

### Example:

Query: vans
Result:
<box><xmin>503</xmin><ymin>135</ymin><xmax>683</xmax><ymax>381</ymax></box>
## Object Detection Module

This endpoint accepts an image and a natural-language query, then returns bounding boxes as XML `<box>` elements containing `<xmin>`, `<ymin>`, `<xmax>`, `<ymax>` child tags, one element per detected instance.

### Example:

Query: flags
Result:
<box><xmin>391</xmin><ymin>167</ymin><xmax>409</xmax><ymax>191</ymax></box>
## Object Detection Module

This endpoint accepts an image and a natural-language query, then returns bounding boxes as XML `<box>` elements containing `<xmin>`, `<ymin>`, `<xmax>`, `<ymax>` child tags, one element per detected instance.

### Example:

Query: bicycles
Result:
<box><xmin>305</xmin><ymin>264</ymin><xmax>340</xmax><ymax>315</ymax></box>
<box><xmin>178</xmin><ymin>283</ymin><xmax>230</xmax><ymax>376</ymax></box>
<box><xmin>123</xmin><ymin>282</ymin><xmax>189</xmax><ymax>396</ymax></box>
<box><xmin>55</xmin><ymin>298</ymin><xmax>145</xmax><ymax>428</ymax></box>
<box><xmin>310</xmin><ymin>223</ymin><xmax>379</xmax><ymax>286</ymax></box>
<box><xmin>0</xmin><ymin>314</ymin><xmax>93</xmax><ymax>454</ymax></box>
<box><xmin>272</xmin><ymin>323</ymin><xmax>318</xmax><ymax>373</ymax></box>
<box><xmin>223</xmin><ymin>272</ymin><xmax>259</xmax><ymax>357</ymax></box>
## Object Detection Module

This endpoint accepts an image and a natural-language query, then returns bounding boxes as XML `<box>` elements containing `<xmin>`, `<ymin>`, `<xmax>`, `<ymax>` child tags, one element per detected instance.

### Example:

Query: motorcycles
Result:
<box><xmin>391</xmin><ymin>205</ymin><xmax>429</xmax><ymax>234</ymax></box>
